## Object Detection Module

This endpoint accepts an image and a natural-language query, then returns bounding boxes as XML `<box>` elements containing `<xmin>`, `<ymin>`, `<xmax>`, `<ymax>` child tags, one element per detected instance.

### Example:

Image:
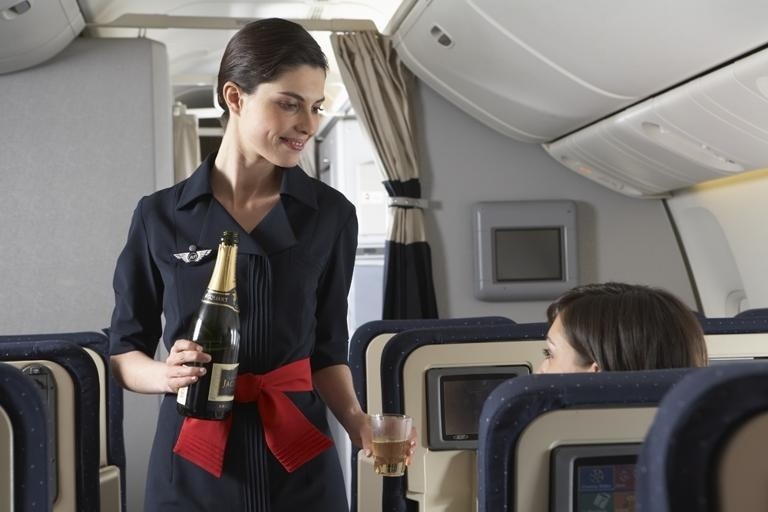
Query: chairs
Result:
<box><xmin>340</xmin><ymin>307</ymin><xmax>768</xmax><ymax>511</ymax></box>
<box><xmin>1</xmin><ymin>330</ymin><xmax>130</xmax><ymax>511</ymax></box>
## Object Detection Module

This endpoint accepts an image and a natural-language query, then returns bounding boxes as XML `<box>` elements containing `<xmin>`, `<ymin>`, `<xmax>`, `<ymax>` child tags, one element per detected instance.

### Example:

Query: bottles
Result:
<box><xmin>174</xmin><ymin>230</ymin><xmax>243</xmax><ymax>424</ymax></box>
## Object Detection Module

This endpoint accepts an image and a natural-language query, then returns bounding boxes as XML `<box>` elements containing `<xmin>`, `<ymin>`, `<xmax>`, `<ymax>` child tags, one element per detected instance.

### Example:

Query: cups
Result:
<box><xmin>368</xmin><ymin>412</ymin><xmax>413</xmax><ymax>478</ymax></box>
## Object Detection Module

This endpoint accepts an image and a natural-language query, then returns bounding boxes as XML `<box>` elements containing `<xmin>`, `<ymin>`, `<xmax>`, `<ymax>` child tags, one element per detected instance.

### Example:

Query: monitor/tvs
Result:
<box><xmin>550</xmin><ymin>444</ymin><xmax>639</xmax><ymax>512</ymax></box>
<box><xmin>472</xmin><ymin>200</ymin><xmax>577</xmax><ymax>301</ymax></box>
<box><xmin>426</xmin><ymin>365</ymin><xmax>530</xmax><ymax>449</ymax></box>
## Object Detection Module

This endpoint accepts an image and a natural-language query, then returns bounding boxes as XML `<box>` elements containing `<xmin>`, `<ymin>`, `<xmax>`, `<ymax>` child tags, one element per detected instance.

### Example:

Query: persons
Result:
<box><xmin>535</xmin><ymin>282</ymin><xmax>709</xmax><ymax>374</ymax></box>
<box><xmin>108</xmin><ymin>18</ymin><xmax>418</xmax><ymax>511</ymax></box>
